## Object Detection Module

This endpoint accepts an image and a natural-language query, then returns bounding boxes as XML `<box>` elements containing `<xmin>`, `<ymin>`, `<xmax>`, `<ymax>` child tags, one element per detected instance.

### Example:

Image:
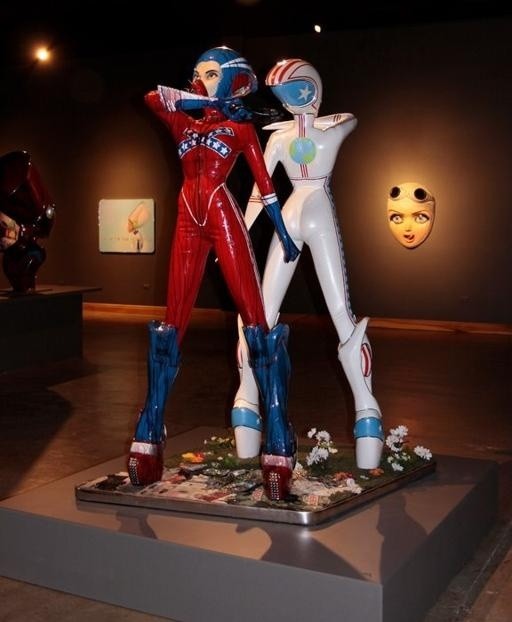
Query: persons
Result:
<box><xmin>214</xmin><ymin>58</ymin><xmax>384</xmax><ymax>469</ymax></box>
<box><xmin>127</xmin><ymin>46</ymin><xmax>300</xmax><ymax>500</ymax></box>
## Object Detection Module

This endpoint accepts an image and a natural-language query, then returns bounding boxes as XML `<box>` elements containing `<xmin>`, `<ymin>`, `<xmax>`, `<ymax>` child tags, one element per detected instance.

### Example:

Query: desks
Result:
<box><xmin>0</xmin><ymin>282</ymin><xmax>102</xmax><ymax>372</ymax></box>
